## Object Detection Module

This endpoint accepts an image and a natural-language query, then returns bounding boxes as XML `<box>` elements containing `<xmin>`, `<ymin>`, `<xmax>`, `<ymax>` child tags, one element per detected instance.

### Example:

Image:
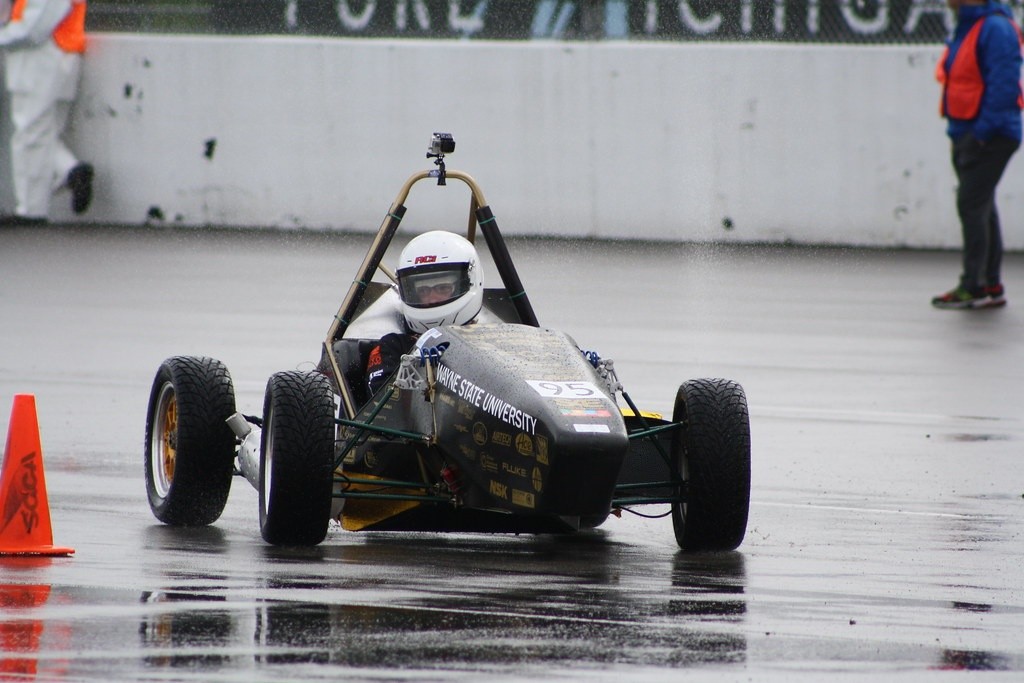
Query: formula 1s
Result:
<box><xmin>144</xmin><ymin>135</ymin><xmax>751</xmax><ymax>551</ymax></box>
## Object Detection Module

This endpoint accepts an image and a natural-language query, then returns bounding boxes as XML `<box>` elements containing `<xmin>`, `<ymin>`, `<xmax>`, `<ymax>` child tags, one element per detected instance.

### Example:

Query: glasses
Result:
<box><xmin>416</xmin><ymin>282</ymin><xmax>453</xmax><ymax>297</ymax></box>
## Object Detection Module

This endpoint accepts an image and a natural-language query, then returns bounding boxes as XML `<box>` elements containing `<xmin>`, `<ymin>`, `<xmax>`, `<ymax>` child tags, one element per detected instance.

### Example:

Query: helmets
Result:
<box><xmin>395</xmin><ymin>231</ymin><xmax>485</xmax><ymax>334</ymax></box>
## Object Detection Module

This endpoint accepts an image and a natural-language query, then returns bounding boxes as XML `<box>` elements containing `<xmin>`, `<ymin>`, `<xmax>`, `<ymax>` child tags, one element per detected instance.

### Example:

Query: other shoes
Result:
<box><xmin>985</xmin><ymin>283</ymin><xmax>1007</xmax><ymax>306</ymax></box>
<box><xmin>0</xmin><ymin>213</ymin><xmax>49</xmax><ymax>229</ymax></box>
<box><xmin>932</xmin><ymin>278</ymin><xmax>993</xmax><ymax>308</ymax></box>
<box><xmin>69</xmin><ymin>163</ymin><xmax>93</xmax><ymax>213</ymax></box>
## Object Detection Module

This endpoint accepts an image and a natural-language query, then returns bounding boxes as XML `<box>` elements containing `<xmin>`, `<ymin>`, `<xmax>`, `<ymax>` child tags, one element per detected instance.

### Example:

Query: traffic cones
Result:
<box><xmin>0</xmin><ymin>554</ymin><xmax>75</xmax><ymax>683</ymax></box>
<box><xmin>1</xmin><ymin>394</ymin><xmax>76</xmax><ymax>554</ymax></box>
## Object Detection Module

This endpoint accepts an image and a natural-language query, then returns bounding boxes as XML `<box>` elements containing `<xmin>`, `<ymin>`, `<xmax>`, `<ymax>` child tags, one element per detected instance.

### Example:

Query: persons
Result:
<box><xmin>363</xmin><ymin>230</ymin><xmax>486</xmax><ymax>400</ymax></box>
<box><xmin>0</xmin><ymin>0</ymin><xmax>98</xmax><ymax>227</ymax></box>
<box><xmin>931</xmin><ymin>0</ymin><xmax>1024</xmax><ymax>307</ymax></box>
